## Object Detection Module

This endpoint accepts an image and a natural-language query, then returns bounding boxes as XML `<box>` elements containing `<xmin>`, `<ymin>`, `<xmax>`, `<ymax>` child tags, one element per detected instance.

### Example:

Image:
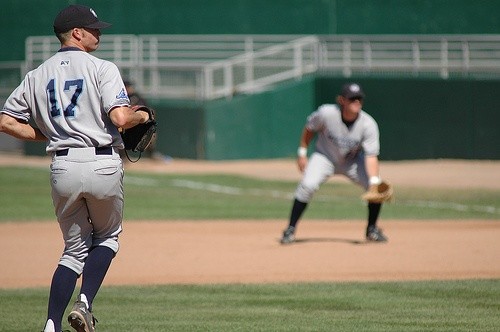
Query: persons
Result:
<box><xmin>280</xmin><ymin>83</ymin><xmax>387</xmax><ymax>243</ymax></box>
<box><xmin>124</xmin><ymin>82</ymin><xmax>145</xmax><ymax>106</ymax></box>
<box><xmin>0</xmin><ymin>5</ymin><xmax>156</xmax><ymax>332</ymax></box>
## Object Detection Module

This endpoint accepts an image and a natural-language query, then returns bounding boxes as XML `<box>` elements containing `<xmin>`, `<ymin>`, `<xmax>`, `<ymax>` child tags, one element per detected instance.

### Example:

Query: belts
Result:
<box><xmin>56</xmin><ymin>146</ymin><xmax>112</xmax><ymax>155</ymax></box>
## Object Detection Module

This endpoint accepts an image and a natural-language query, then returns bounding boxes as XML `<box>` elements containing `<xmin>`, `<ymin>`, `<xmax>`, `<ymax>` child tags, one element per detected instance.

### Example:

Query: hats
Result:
<box><xmin>340</xmin><ymin>82</ymin><xmax>365</xmax><ymax>100</ymax></box>
<box><xmin>54</xmin><ymin>5</ymin><xmax>113</xmax><ymax>33</ymax></box>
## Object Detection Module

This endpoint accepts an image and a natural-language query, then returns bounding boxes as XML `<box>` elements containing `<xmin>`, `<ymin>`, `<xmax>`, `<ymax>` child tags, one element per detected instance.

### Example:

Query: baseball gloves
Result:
<box><xmin>359</xmin><ymin>180</ymin><xmax>397</xmax><ymax>203</ymax></box>
<box><xmin>117</xmin><ymin>104</ymin><xmax>158</xmax><ymax>153</ymax></box>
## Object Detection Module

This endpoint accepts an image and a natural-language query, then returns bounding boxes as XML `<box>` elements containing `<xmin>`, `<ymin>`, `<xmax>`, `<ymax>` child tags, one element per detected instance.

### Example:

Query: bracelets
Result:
<box><xmin>297</xmin><ymin>147</ymin><xmax>308</xmax><ymax>156</ymax></box>
<box><xmin>368</xmin><ymin>177</ymin><xmax>379</xmax><ymax>185</ymax></box>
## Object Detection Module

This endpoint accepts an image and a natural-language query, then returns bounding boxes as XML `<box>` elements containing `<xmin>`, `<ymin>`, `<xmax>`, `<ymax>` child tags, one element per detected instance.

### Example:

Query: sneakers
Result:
<box><xmin>365</xmin><ymin>227</ymin><xmax>384</xmax><ymax>242</ymax></box>
<box><xmin>282</xmin><ymin>225</ymin><xmax>295</xmax><ymax>243</ymax></box>
<box><xmin>67</xmin><ymin>296</ymin><xmax>98</xmax><ymax>332</ymax></box>
